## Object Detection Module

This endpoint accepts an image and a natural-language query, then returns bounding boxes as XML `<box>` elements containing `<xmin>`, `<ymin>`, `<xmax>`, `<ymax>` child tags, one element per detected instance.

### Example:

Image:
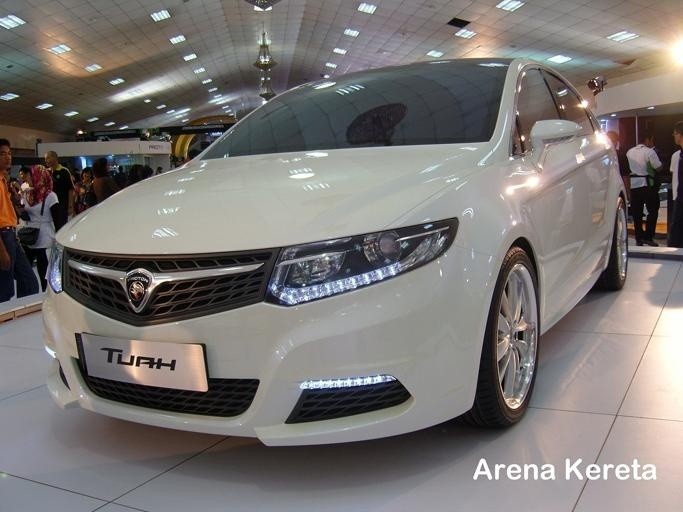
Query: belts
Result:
<box><xmin>1</xmin><ymin>227</ymin><xmax>17</xmax><ymax>232</ymax></box>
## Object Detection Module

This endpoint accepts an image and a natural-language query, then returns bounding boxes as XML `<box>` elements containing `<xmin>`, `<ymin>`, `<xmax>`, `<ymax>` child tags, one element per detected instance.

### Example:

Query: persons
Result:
<box><xmin>665</xmin><ymin>120</ymin><xmax>682</xmax><ymax>247</ymax></box>
<box><xmin>668</xmin><ymin>148</ymin><xmax>680</xmax><ymax>228</ymax></box>
<box><xmin>0</xmin><ymin>133</ymin><xmax>201</xmax><ymax>303</ymax></box>
<box><xmin>626</xmin><ymin>128</ymin><xmax>665</xmax><ymax>247</ymax></box>
<box><xmin>606</xmin><ymin>130</ymin><xmax>632</xmax><ymax>205</ymax></box>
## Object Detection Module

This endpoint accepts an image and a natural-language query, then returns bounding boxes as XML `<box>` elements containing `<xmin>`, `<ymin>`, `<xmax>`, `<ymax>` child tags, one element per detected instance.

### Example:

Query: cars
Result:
<box><xmin>41</xmin><ymin>57</ymin><xmax>629</xmax><ymax>447</ymax></box>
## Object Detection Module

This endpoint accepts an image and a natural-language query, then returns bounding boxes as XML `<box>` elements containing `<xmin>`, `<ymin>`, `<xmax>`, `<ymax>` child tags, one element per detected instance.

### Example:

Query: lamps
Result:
<box><xmin>252</xmin><ymin>21</ymin><xmax>276</xmax><ymax>101</ymax></box>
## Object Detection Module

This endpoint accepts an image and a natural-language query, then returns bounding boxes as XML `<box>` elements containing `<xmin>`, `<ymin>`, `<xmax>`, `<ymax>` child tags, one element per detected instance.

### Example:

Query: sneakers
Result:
<box><xmin>636</xmin><ymin>240</ymin><xmax>643</xmax><ymax>246</ymax></box>
<box><xmin>643</xmin><ymin>238</ymin><xmax>658</xmax><ymax>246</ymax></box>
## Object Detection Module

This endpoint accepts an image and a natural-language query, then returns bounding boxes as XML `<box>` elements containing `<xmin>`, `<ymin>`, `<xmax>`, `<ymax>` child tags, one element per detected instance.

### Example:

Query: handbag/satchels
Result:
<box><xmin>18</xmin><ymin>227</ymin><xmax>40</xmax><ymax>244</ymax></box>
